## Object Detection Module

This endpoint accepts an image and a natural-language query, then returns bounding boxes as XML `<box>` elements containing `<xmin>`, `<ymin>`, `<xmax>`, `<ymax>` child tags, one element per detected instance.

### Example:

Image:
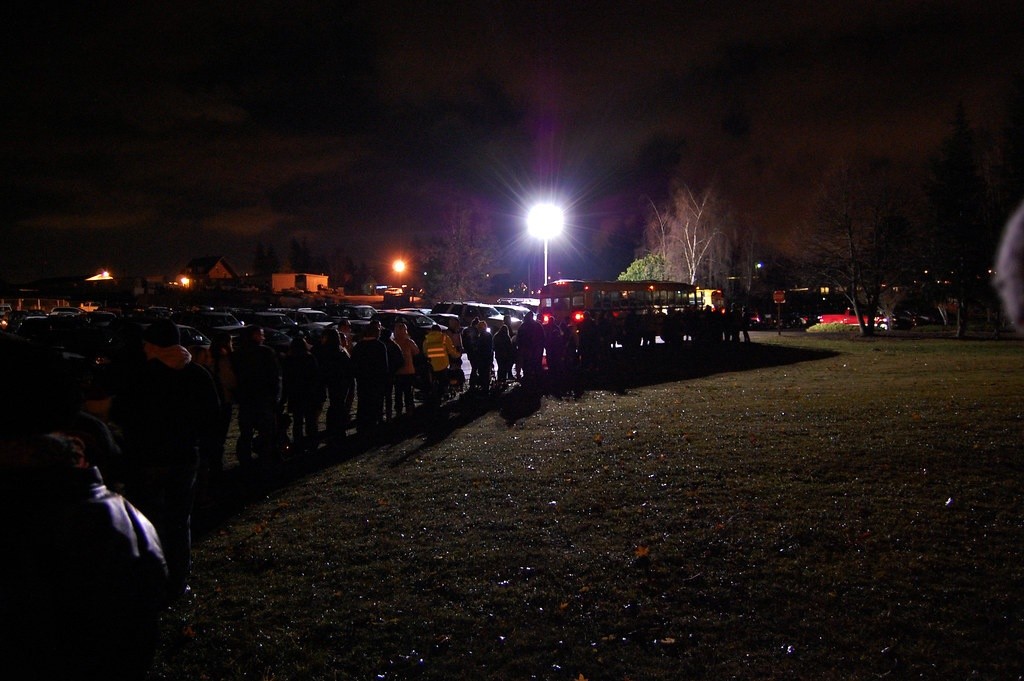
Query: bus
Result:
<box><xmin>538</xmin><ymin>275</ymin><xmax>704</xmax><ymax>349</ymax></box>
<box><xmin>675</xmin><ymin>287</ymin><xmax>727</xmax><ymax>316</ymax></box>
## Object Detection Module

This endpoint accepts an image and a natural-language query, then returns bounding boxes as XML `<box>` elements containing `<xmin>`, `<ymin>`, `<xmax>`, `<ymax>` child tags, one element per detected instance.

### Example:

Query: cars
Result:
<box><xmin>746</xmin><ymin>311</ymin><xmax>768</xmax><ymax>330</ymax></box>
<box><xmin>816</xmin><ymin>305</ymin><xmax>897</xmax><ymax>332</ymax></box>
<box><xmin>383</xmin><ymin>286</ymin><xmax>405</xmax><ymax>298</ymax></box>
<box><xmin>0</xmin><ymin>295</ymin><xmax>540</xmax><ymax>368</ymax></box>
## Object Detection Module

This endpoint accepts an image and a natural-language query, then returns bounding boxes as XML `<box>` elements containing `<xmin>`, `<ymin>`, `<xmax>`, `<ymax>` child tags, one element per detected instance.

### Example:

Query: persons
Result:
<box><xmin>392</xmin><ymin>323</ymin><xmax>420</xmax><ymax>415</ymax></box>
<box><xmin>0</xmin><ymin>304</ymin><xmax>754</xmax><ymax>681</ymax></box>
<box><xmin>422</xmin><ymin>325</ymin><xmax>463</xmax><ymax>394</ymax></box>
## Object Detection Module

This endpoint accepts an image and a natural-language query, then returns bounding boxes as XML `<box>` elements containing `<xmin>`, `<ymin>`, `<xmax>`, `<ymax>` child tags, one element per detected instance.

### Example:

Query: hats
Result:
<box><xmin>141</xmin><ymin>319</ymin><xmax>180</xmax><ymax>347</ymax></box>
<box><xmin>367</xmin><ymin>319</ymin><xmax>383</xmax><ymax>331</ymax></box>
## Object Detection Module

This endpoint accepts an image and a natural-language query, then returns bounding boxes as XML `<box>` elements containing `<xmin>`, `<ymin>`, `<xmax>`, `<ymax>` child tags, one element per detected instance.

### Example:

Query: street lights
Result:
<box><xmin>520</xmin><ymin>192</ymin><xmax>571</xmax><ymax>308</ymax></box>
<box><xmin>754</xmin><ymin>264</ymin><xmax>762</xmax><ymax>282</ymax></box>
<box><xmin>392</xmin><ymin>259</ymin><xmax>408</xmax><ymax>288</ymax></box>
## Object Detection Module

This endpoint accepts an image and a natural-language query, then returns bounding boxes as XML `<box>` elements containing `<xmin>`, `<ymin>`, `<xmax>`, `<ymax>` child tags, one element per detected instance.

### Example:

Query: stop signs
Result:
<box><xmin>772</xmin><ymin>291</ymin><xmax>786</xmax><ymax>304</ymax></box>
